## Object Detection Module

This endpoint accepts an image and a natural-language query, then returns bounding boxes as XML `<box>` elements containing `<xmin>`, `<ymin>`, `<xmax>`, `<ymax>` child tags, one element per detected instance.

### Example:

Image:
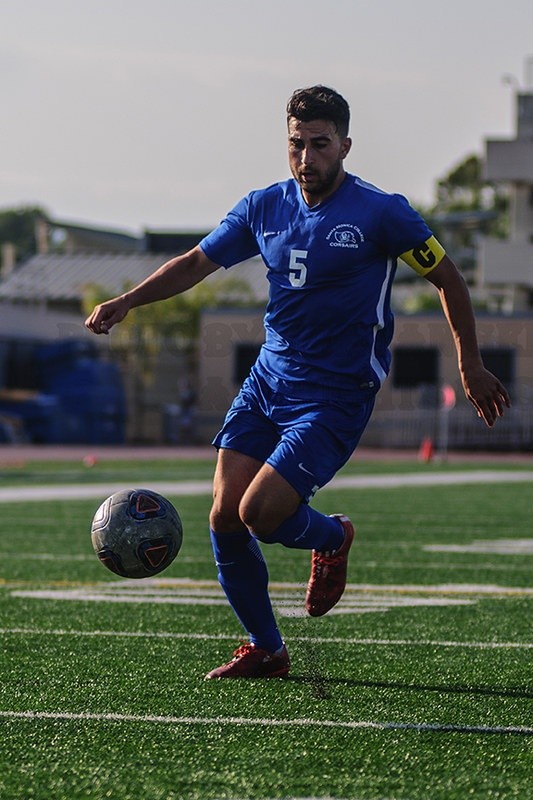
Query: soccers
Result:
<box><xmin>91</xmin><ymin>487</ymin><xmax>183</xmax><ymax>579</ymax></box>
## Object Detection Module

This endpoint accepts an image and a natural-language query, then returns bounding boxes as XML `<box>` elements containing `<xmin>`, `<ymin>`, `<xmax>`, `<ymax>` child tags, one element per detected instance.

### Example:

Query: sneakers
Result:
<box><xmin>203</xmin><ymin>638</ymin><xmax>292</xmax><ymax>679</ymax></box>
<box><xmin>305</xmin><ymin>513</ymin><xmax>354</xmax><ymax>617</ymax></box>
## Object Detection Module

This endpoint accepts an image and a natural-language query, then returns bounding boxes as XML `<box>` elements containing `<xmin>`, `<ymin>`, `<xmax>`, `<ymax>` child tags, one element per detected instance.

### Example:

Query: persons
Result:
<box><xmin>82</xmin><ymin>84</ymin><xmax>511</xmax><ymax>679</ymax></box>
